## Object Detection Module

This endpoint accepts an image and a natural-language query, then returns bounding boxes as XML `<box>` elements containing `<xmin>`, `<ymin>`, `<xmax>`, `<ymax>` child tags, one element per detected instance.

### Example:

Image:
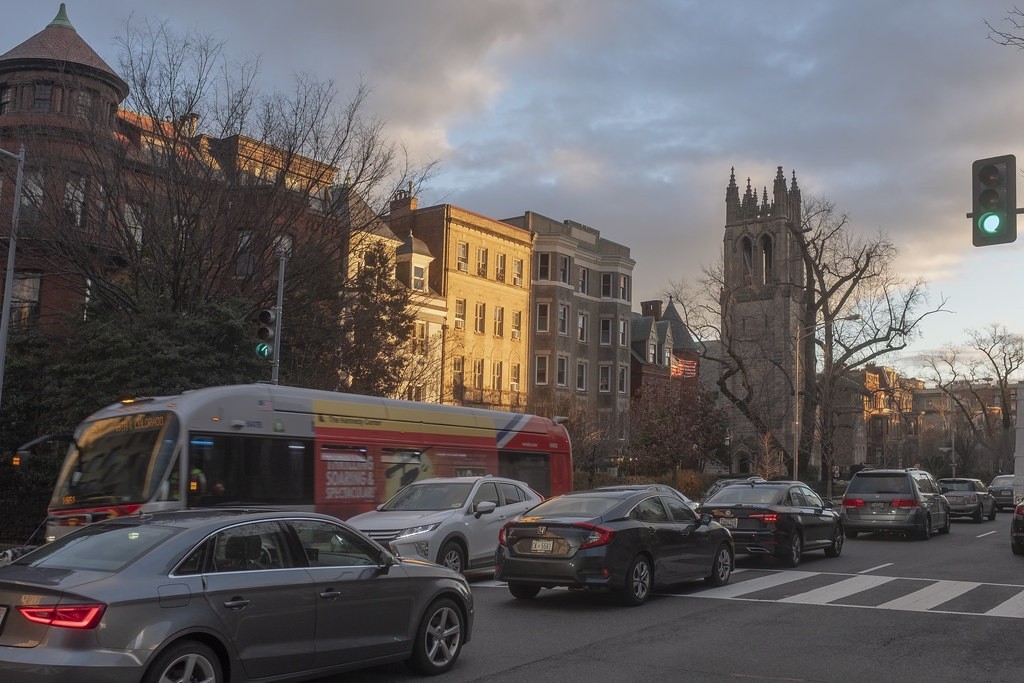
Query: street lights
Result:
<box><xmin>950</xmin><ymin>378</ymin><xmax>993</xmax><ymax>478</ymax></box>
<box><xmin>792</xmin><ymin>314</ymin><xmax>863</xmax><ymax>481</ymax></box>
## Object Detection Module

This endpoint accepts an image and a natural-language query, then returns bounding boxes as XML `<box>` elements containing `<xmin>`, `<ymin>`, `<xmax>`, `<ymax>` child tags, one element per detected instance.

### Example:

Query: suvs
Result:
<box><xmin>840</xmin><ymin>467</ymin><xmax>951</xmax><ymax>540</ymax></box>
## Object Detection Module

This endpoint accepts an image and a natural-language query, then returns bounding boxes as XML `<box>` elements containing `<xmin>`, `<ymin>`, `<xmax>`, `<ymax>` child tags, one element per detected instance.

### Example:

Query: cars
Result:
<box><xmin>0</xmin><ymin>509</ymin><xmax>474</xmax><ymax>683</ymax></box>
<box><xmin>988</xmin><ymin>474</ymin><xmax>1014</xmax><ymax>511</ymax></box>
<box><xmin>598</xmin><ymin>475</ymin><xmax>767</xmax><ymax>511</ymax></box>
<box><xmin>694</xmin><ymin>479</ymin><xmax>844</xmax><ymax>567</ymax></box>
<box><xmin>938</xmin><ymin>478</ymin><xmax>997</xmax><ymax>523</ymax></box>
<box><xmin>1010</xmin><ymin>493</ymin><xmax>1024</xmax><ymax>553</ymax></box>
<box><xmin>494</xmin><ymin>491</ymin><xmax>735</xmax><ymax>604</ymax></box>
<box><xmin>329</xmin><ymin>474</ymin><xmax>546</xmax><ymax>574</ymax></box>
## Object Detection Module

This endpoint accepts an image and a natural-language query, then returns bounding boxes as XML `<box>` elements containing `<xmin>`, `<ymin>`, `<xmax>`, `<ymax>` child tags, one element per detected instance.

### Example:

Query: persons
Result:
<box><xmin>169</xmin><ymin>445</ymin><xmax>208</xmax><ymax>502</ymax></box>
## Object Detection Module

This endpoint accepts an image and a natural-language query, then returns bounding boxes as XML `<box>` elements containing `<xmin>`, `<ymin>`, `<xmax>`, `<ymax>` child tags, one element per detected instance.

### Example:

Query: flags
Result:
<box><xmin>671</xmin><ymin>355</ymin><xmax>696</xmax><ymax>377</ymax></box>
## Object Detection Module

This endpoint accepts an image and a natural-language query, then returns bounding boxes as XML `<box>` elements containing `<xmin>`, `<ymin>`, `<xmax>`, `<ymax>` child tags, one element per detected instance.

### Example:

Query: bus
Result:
<box><xmin>10</xmin><ymin>382</ymin><xmax>574</xmax><ymax>547</ymax></box>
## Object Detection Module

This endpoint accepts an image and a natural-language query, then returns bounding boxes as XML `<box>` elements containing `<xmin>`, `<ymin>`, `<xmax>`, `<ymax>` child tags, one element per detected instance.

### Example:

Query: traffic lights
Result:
<box><xmin>255</xmin><ymin>309</ymin><xmax>277</xmax><ymax>358</ymax></box>
<box><xmin>972</xmin><ymin>154</ymin><xmax>1017</xmax><ymax>247</ymax></box>
